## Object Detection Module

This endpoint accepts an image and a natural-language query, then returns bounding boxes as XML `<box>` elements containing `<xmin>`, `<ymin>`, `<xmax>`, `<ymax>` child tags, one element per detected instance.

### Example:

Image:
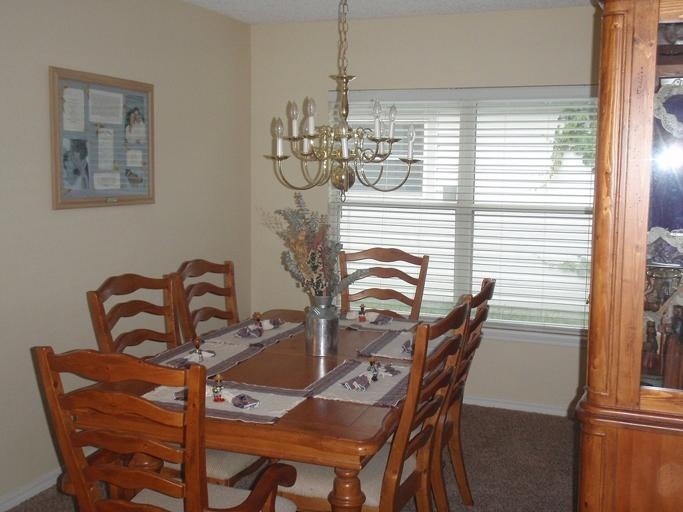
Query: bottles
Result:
<box><xmin>251</xmin><ymin>311</ymin><xmax>264</xmax><ymax>334</ymax></box>
<box><xmin>366</xmin><ymin>357</ymin><xmax>378</xmax><ymax>383</ymax></box>
<box><xmin>192</xmin><ymin>339</ymin><xmax>204</xmax><ymax>363</ymax></box>
<box><xmin>358</xmin><ymin>305</ymin><xmax>367</xmax><ymax>323</ymax></box>
<box><xmin>211</xmin><ymin>373</ymin><xmax>227</xmax><ymax>402</ymax></box>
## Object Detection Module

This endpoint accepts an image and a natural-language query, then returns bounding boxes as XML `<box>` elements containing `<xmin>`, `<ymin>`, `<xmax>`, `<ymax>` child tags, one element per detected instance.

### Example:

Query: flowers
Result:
<box><xmin>257</xmin><ymin>191</ymin><xmax>374</xmax><ymax>301</ymax></box>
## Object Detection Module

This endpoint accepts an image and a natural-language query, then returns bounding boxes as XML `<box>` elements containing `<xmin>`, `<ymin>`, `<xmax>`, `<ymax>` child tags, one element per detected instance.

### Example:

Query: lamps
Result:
<box><xmin>263</xmin><ymin>2</ymin><xmax>423</xmax><ymax>200</ymax></box>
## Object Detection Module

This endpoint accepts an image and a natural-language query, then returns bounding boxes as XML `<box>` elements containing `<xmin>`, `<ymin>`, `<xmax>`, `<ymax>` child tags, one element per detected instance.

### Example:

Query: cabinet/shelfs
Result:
<box><xmin>573</xmin><ymin>0</ymin><xmax>683</xmax><ymax>512</ymax></box>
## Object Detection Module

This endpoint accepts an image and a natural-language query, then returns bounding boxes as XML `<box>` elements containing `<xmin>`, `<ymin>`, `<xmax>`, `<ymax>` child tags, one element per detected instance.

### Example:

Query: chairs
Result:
<box><xmin>23</xmin><ymin>336</ymin><xmax>299</xmax><ymax>512</ymax></box>
<box><xmin>331</xmin><ymin>244</ymin><xmax>434</xmax><ymax>325</ymax></box>
<box><xmin>265</xmin><ymin>296</ymin><xmax>472</xmax><ymax>512</ymax></box>
<box><xmin>388</xmin><ymin>277</ymin><xmax>502</xmax><ymax>508</ymax></box>
<box><xmin>166</xmin><ymin>255</ymin><xmax>247</xmax><ymax>343</ymax></box>
<box><xmin>82</xmin><ymin>272</ymin><xmax>269</xmax><ymax>487</ymax></box>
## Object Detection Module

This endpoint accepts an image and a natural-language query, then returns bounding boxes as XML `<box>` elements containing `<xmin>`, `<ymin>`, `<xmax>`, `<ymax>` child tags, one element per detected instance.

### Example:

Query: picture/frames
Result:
<box><xmin>45</xmin><ymin>64</ymin><xmax>159</xmax><ymax>211</ymax></box>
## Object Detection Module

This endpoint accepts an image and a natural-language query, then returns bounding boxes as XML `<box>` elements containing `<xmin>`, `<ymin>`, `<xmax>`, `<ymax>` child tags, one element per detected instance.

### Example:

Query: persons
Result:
<box><xmin>132</xmin><ymin>108</ymin><xmax>145</xmax><ymax>144</ymax></box>
<box><xmin>124</xmin><ymin>112</ymin><xmax>137</xmax><ymax>144</ymax></box>
